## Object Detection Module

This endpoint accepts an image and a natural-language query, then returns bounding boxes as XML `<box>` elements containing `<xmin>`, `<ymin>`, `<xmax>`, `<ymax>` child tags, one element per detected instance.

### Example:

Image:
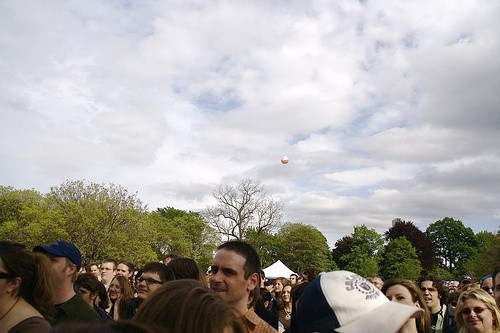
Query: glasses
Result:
<box><xmin>137</xmin><ymin>277</ymin><xmax>164</xmax><ymax>285</ymax></box>
<box><xmin>482</xmin><ymin>287</ymin><xmax>494</xmax><ymax>291</ymax></box>
<box><xmin>460</xmin><ymin>307</ymin><xmax>488</xmax><ymax>314</ymax></box>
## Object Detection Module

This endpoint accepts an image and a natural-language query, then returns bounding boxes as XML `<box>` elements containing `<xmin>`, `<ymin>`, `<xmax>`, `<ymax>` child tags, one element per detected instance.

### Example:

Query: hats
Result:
<box><xmin>291</xmin><ymin>270</ymin><xmax>425</xmax><ymax>332</ymax></box>
<box><xmin>31</xmin><ymin>241</ymin><xmax>81</xmax><ymax>272</ymax></box>
<box><xmin>461</xmin><ymin>275</ymin><xmax>472</xmax><ymax>283</ymax></box>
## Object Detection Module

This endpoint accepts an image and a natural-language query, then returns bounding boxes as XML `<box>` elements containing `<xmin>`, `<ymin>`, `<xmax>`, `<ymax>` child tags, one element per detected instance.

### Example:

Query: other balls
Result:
<box><xmin>281</xmin><ymin>156</ymin><xmax>288</xmax><ymax>164</ymax></box>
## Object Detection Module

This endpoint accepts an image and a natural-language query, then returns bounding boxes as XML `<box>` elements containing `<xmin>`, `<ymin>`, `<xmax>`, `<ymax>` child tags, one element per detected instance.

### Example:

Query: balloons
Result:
<box><xmin>281</xmin><ymin>156</ymin><xmax>289</xmax><ymax>164</ymax></box>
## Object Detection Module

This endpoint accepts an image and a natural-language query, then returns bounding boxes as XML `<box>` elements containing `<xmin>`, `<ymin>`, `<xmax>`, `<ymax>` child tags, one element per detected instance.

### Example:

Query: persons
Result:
<box><xmin>1</xmin><ymin>239</ymin><xmax>500</xmax><ymax>333</ymax></box>
